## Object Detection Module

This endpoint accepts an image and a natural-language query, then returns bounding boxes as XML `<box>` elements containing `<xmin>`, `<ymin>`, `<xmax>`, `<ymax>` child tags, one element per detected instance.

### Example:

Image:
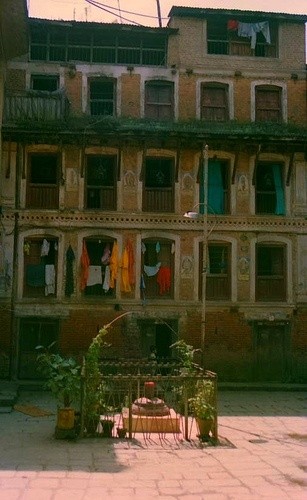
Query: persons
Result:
<box><xmin>143</xmin><ymin>346</ymin><xmax>159</xmax><ymax>375</ymax></box>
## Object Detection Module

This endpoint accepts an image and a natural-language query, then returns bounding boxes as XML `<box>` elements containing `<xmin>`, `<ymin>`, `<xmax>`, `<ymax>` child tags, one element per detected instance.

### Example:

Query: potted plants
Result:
<box><xmin>188</xmin><ymin>379</ymin><xmax>215</xmax><ymax>442</ymax></box>
<box><xmin>38</xmin><ymin>340</ymin><xmax>131</xmax><ymax>443</ymax></box>
